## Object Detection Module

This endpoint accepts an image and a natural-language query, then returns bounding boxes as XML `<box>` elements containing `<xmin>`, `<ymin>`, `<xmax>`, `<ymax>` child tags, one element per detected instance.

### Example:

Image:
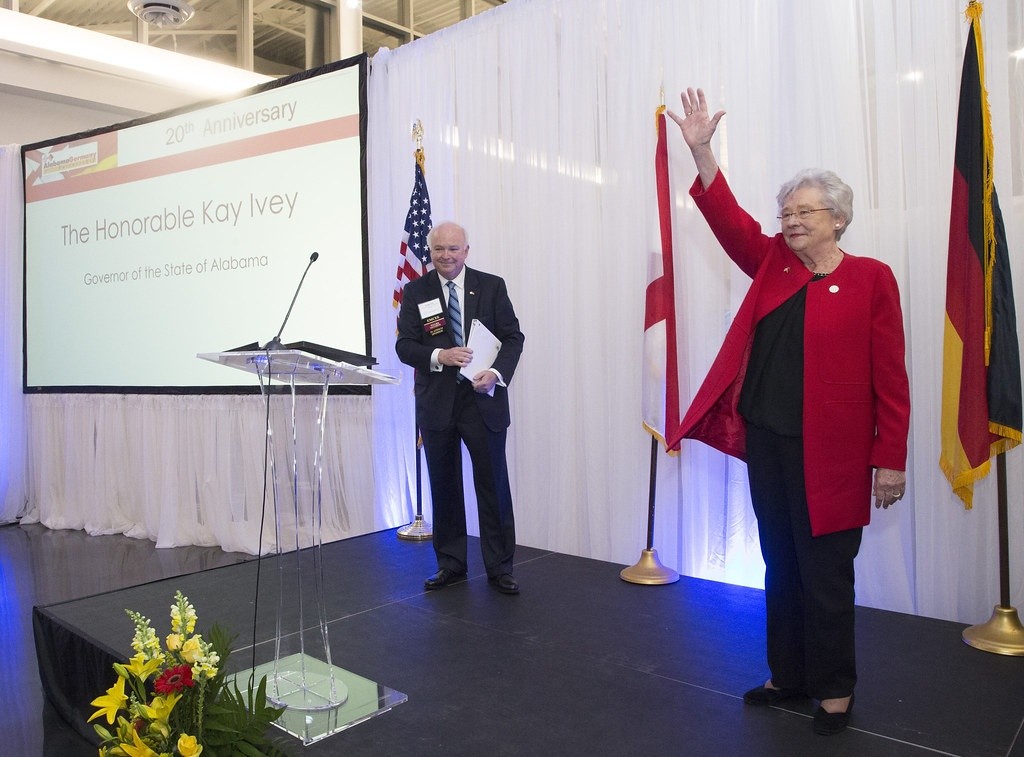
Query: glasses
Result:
<box><xmin>777</xmin><ymin>205</ymin><xmax>831</xmax><ymax>218</ymax></box>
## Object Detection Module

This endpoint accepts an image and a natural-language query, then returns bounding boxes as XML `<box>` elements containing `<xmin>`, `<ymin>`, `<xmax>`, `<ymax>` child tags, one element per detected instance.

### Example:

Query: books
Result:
<box><xmin>459</xmin><ymin>318</ymin><xmax>502</xmax><ymax>398</ymax></box>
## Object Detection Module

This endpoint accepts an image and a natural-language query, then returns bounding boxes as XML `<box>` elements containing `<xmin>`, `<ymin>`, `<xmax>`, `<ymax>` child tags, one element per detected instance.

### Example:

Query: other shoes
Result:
<box><xmin>742</xmin><ymin>681</ymin><xmax>811</xmax><ymax>706</ymax></box>
<box><xmin>813</xmin><ymin>679</ymin><xmax>856</xmax><ymax>736</ymax></box>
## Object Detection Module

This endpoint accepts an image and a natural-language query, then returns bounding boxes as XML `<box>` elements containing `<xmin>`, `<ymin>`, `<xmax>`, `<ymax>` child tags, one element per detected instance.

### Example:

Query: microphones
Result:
<box><xmin>260</xmin><ymin>252</ymin><xmax>319</xmax><ymax>350</ymax></box>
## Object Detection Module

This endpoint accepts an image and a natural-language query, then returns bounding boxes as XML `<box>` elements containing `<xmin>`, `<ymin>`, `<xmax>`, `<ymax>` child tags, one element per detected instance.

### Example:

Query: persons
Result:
<box><xmin>394</xmin><ymin>222</ymin><xmax>525</xmax><ymax>594</ymax></box>
<box><xmin>666</xmin><ymin>87</ymin><xmax>911</xmax><ymax>736</ymax></box>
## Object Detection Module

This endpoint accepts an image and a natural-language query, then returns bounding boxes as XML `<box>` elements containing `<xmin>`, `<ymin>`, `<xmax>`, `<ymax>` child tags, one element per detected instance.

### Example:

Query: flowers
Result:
<box><xmin>87</xmin><ymin>590</ymin><xmax>298</xmax><ymax>757</ymax></box>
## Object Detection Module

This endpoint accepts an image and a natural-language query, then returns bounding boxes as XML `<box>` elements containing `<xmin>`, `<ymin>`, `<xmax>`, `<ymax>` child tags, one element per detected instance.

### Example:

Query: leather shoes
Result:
<box><xmin>426</xmin><ymin>566</ymin><xmax>469</xmax><ymax>590</ymax></box>
<box><xmin>488</xmin><ymin>571</ymin><xmax>520</xmax><ymax>595</ymax></box>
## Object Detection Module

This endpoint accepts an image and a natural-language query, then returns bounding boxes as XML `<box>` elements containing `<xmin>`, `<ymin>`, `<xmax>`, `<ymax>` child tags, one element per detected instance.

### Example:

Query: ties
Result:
<box><xmin>444</xmin><ymin>281</ymin><xmax>466</xmax><ymax>345</ymax></box>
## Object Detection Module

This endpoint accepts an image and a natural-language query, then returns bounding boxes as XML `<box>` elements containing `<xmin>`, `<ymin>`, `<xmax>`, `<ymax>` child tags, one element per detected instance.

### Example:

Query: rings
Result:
<box><xmin>686</xmin><ymin>110</ymin><xmax>693</xmax><ymax>116</ymax></box>
<box><xmin>893</xmin><ymin>493</ymin><xmax>900</xmax><ymax>498</ymax></box>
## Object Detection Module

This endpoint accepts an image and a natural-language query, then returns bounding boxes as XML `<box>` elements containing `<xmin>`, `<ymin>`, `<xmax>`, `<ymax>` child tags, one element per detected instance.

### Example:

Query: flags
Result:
<box><xmin>391</xmin><ymin>150</ymin><xmax>434</xmax><ymax>336</ymax></box>
<box><xmin>638</xmin><ymin>111</ymin><xmax>692</xmax><ymax>457</ymax></box>
<box><xmin>937</xmin><ymin>0</ymin><xmax>1024</xmax><ymax>510</ymax></box>
